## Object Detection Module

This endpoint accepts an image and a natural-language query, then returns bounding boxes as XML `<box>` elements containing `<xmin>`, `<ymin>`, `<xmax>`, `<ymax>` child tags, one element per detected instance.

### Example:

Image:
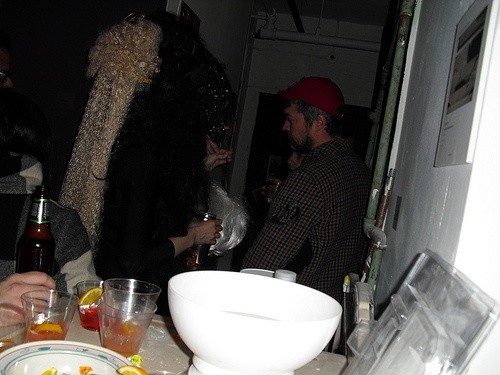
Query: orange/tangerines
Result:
<box><xmin>34</xmin><ymin>323</ymin><xmax>62</xmax><ymax>332</ymax></box>
<box><xmin>116</xmin><ymin>365</ymin><xmax>149</xmax><ymax>375</ymax></box>
<box><xmin>80</xmin><ymin>287</ymin><xmax>103</xmax><ymax>304</ymax></box>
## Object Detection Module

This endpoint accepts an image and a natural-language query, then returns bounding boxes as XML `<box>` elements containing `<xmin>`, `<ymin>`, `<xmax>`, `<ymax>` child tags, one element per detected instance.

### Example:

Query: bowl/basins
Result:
<box><xmin>167</xmin><ymin>270</ymin><xmax>343</xmax><ymax>374</ymax></box>
<box><xmin>0</xmin><ymin>339</ymin><xmax>135</xmax><ymax>375</ymax></box>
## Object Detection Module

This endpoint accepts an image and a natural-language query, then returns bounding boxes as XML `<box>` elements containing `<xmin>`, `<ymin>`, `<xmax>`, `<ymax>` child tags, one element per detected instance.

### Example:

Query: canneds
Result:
<box><xmin>185</xmin><ymin>210</ymin><xmax>216</xmax><ymax>270</ymax></box>
<box><xmin>268</xmin><ymin>178</ymin><xmax>282</xmax><ymax>202</ymax></box>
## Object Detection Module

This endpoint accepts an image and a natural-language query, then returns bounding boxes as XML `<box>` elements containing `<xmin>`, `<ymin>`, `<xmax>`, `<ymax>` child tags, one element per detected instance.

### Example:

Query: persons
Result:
<box><xmin>100</xmin><ymin>76</ymin><xmax>371</xmax><ymax>305</ymax></box>
<box><xmin>0</xmin><ymin>88</ymin><xmax>99</xmax><ymax>326</ymax></box>
<box><xmin>0</xmin><ymin>43</ymin><xmax>18</xmax><ymax>89</ymax></box>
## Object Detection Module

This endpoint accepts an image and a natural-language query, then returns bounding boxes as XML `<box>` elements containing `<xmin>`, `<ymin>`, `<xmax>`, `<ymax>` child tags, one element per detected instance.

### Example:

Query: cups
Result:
<box><xmin>20</xmin><ymin>289</ymin><xmax>80</xmax><ymax>343</ymax></box>
<box><xmin>74</xmin><ymin>280</ymin><xmax>105</xmax><ymax>332</ymax></box>
<box><xmin>265</xmin><ymin>175</ymin><xmax>285</xmax><ymax>208</ymax></box>
<box><xmin>97</xmin><ymin>291</ymin><xmax>157</xmax><ymax>358</ymax></box>
<box><xmin>183</xmin><ymin>210</ymin><xmax>217</xmax><ymax>271</ymax></box>
<box><xmin>0</xmin><ymin>303</ymin><xmax>31</xmax><ymax>352</ymax></box>
<box><xmin>102</xmin><ymin>277</ymin><xmax>162</xmax><ymax>313</ymax></box>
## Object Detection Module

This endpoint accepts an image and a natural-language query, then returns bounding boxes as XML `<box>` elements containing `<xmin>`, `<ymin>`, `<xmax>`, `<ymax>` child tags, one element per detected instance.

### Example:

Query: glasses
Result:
<box><xmin>0</xmin><ymin>69</ymin><xmax>15</xmax><ymax>84</ymax></box>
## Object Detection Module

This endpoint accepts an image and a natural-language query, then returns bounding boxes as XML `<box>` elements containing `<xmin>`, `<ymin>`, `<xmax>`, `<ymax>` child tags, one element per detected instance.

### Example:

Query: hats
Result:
<box><xmin>280</xmin><ymin>77</ymin><xmax>344</xmax><ymax>121</ymax></box>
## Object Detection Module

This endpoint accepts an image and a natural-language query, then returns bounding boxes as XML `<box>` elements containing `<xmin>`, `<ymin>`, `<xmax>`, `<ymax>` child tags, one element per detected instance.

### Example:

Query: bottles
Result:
<box><xmin>15</xmin><ymin>184</ymin><xmax>57</xmax><ymax>277</ymax></box>
